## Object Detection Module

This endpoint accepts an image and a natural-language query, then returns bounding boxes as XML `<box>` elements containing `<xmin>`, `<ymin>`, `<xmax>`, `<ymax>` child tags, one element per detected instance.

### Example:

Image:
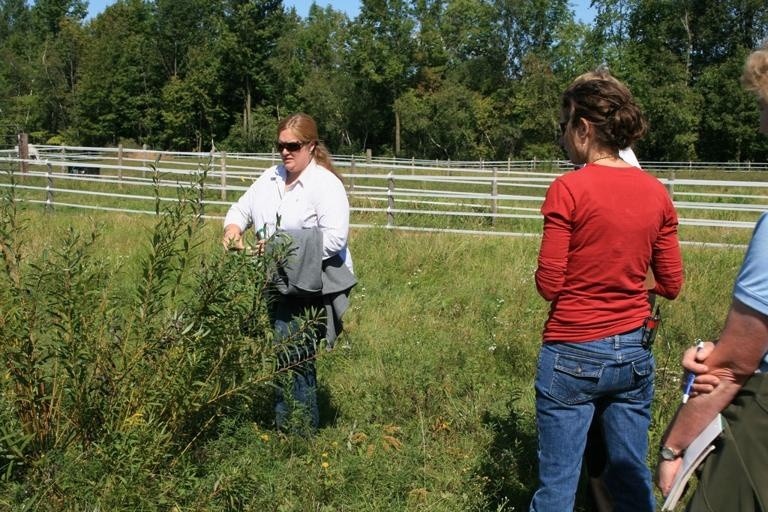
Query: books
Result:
<box><xmin>660</xmin><ymin>413</ymin><xmax>722</xmax><ymax>512</ymax></box>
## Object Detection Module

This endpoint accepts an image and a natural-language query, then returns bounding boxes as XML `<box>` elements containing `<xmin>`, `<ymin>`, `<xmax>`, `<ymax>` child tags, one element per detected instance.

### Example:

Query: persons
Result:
<box><xmin>531</xmin><ymin>70</ymin><xmax>684</xmax><ymax>512</ymax></box>
<box><xmin>223</xmin><ymin>113</ymin><xmax>354</xmax><ymax>436</ymax></box>
<box><xmin>654</xmin><ymin>40</ymin><xmax>768</xmax><ymax>512</ymax></box>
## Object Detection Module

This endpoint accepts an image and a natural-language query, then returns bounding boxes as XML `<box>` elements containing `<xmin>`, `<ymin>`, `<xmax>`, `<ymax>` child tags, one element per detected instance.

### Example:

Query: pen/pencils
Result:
<box><xmin>682</xmin><ymin>340</ymin><xmax>704</xmax><ymax>404</ymax></box>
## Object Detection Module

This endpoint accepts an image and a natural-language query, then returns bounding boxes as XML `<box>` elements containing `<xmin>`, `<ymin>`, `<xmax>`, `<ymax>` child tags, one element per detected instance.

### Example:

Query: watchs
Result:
<box><xmin>659</xmin><ymin>445</ymin><xmax>683</xmax><ymax>460</ymax></box>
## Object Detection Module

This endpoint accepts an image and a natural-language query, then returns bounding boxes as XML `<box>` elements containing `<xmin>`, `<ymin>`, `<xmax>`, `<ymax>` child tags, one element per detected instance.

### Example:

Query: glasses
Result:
<box><xmin>277</xmin><ymin>141</ymin><xmax>311</xmax><ymax>151</ymax></box>
